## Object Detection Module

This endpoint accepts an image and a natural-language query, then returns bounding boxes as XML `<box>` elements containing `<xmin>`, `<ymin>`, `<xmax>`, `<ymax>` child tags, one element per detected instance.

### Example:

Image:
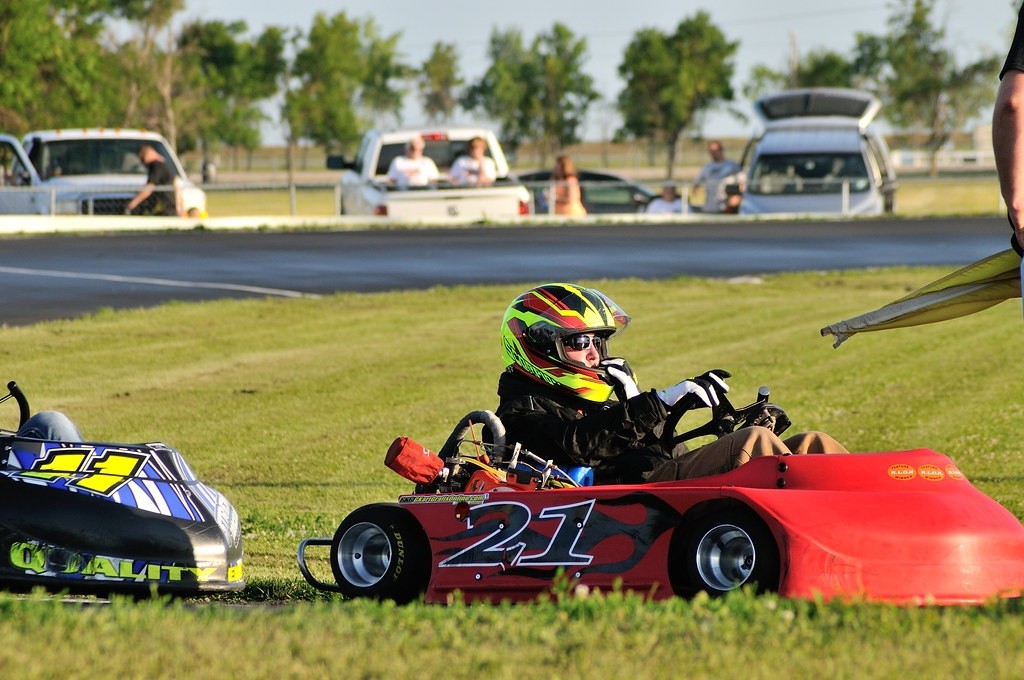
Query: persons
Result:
<box><xmin>646</xmin><ymin>183</ymin><xmax>694</xmax><ymax>213</ymax></box>
<box><xmin>481</xmin><ymin>283</ymin><xmax>849</xmax><ymax>486</ymax></box>
<box><xmin>992</xmin><ymin>0</ymin><xmax>1024</xmax><ymax>309</ymax></box>
<box><xmin>543</xmin><ymin>156</ymin><xmax>587</xmax><ymax>214</ymax></box>
<box><xmin>125</xmin><ymin>143</ymin><xmax>183</xmax><ymax>216</ymax></box>
<box><xmin>448</xmin><ymin>137</ymin><xmax>496</xmax><ymax>188</ymax></box>
<box><xmin>386</xmin><ymin>134</ymin><xmax>440</xmax><ymax>190</ymax></box>
<box><xmin>693</xmin><ymin>140</ymin><xmax>740</xmax><ymax>213</ymax></box>
<box><xmin>17</xmin><ymin>411</ymin><xmax>81</xmax><ymax>442</ymax></box>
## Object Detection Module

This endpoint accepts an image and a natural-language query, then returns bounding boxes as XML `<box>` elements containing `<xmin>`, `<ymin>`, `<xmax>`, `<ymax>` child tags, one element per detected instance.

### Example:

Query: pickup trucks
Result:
<box><xmin>327</xmin><ymin>126</ymin><xmax>535</xmax><ymax>215</ymax></box>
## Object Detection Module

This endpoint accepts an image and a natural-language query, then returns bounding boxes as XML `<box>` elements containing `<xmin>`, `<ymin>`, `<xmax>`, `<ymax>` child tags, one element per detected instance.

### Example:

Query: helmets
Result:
<box><xmin>500</xmin><ymin>282</ymin><xmax>632</xmax><ymax>414</ymax></box>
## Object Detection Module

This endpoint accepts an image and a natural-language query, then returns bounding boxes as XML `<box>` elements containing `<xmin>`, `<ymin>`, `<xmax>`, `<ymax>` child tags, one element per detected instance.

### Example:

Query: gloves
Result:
<box><xmin>597</xmin><ymin>357</ymin><xmax>641</xmax><ymax>401</ymax></box>
<box><xmin>652</xmin><ymin>369</ymin><xmax>732</xmax><ymax>413</ymax></box>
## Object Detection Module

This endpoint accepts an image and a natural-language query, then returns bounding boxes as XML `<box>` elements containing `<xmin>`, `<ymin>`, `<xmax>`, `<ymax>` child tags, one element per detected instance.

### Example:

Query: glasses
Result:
<box><xmin>561</xmin><ymin>335</ymin><xmax>602</xmax><ymax>351</ymax></box>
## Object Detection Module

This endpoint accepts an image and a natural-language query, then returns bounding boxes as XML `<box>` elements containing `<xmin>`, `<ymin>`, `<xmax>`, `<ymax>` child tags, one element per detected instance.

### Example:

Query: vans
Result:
<box><xmin>738</xmin><ymin>88</ymin><xmax>899</xmax><ymax>213</ymax></box>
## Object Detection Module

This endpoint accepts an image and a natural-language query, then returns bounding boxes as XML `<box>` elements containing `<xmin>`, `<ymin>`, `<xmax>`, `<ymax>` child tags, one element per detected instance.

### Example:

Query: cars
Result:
<box><xmin>0</xmin><ymin>129</ymin><xmax>206</xmax><ymax>216</ymax></box>
<box><xmin>518</xmin><ymin>170</ymin><xmax>656</xmax><ymax>214</ymax></box>
